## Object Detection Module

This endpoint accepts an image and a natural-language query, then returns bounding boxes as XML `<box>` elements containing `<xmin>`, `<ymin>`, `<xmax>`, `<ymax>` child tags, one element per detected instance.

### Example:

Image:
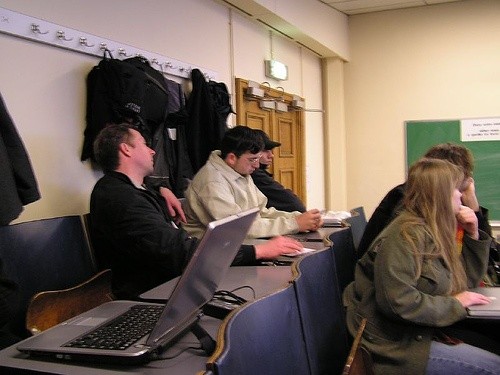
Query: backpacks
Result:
<box><xmin>164</xmin><ymin>77</ymin><xmax>186</xmax><ymax>116</ymax></box>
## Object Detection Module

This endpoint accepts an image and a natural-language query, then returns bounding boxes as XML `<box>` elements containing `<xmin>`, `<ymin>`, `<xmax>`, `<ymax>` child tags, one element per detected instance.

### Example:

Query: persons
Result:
<box><xmin>89</xmin><ymin>123</ymin><xmax>303</xmax><ymax>300</ymax></box>
<box><xmin>356</xmin><ymin>141</ymin><xmax>500</xmax><ymax>287</ymax></box>
<box><xmin>181</xmin><ymin>126</ymin><xmax>324</xmax><ymax>237</ymax></box>
<box><xmin>250</xmin><ymin>129</ymin><xmax>306</xmax><ymax>214</ymax></box>
<box><xmin>342</xmin><ymin>158</ymin><xmax>500</xmax><ymax>375</ymax></box>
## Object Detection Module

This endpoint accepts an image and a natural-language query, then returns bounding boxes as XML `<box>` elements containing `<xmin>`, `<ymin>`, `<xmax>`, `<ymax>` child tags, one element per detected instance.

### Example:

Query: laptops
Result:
<box><xmin>17</xmin><ymin>208</ymin><xmax>261</xmax><ymax>365</ymax></box>
<box><xmin>464</xmin><ymin>287</ymin><xmax>500</xmax><ymax>317</ymax></box>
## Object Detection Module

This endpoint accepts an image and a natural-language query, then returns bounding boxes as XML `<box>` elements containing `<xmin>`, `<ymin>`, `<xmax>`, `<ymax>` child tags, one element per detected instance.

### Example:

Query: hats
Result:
<box><xmin>254</xmin><ymin>129</ymin><xmax>281</xmax><ymax>150</ymax></box>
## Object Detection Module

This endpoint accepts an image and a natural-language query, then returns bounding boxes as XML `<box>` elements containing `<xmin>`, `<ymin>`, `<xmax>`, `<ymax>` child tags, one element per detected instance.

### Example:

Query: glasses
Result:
<box><xmin>239</xmin><ymin>153</ymin><xmax>263</xmax><ymax>166</ymax></box>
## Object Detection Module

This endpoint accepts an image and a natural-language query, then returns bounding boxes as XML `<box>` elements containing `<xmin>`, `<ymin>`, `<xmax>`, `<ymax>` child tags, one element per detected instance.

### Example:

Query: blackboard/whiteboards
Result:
<box><xmin>404</xmin><ymin>116</ymin><xmax>500</xmax><ymax>226</ymax></box>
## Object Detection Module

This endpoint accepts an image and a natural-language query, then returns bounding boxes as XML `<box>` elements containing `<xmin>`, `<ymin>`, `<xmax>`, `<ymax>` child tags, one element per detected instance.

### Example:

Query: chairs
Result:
<box><xmin>0</xmin><ymin>197</ymin><xmax>370</xmax><ymax>375</ymax></box>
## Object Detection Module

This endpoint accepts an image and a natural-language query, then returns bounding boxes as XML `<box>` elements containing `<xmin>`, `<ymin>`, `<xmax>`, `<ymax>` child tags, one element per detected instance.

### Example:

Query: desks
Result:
<box><xmin>141</xmin><ymin>240</ymin><xmax>326</xmax><ymax>318</ymax></box>
<box><xmin>0</xmin><ymin>299</ymin><xmax>225</xmax><ymax>375</ymax></box>
<box><xmin>288</xmin><ymin>223</ymin><xmax>350</xmax><ymax>241</ymax></box>
